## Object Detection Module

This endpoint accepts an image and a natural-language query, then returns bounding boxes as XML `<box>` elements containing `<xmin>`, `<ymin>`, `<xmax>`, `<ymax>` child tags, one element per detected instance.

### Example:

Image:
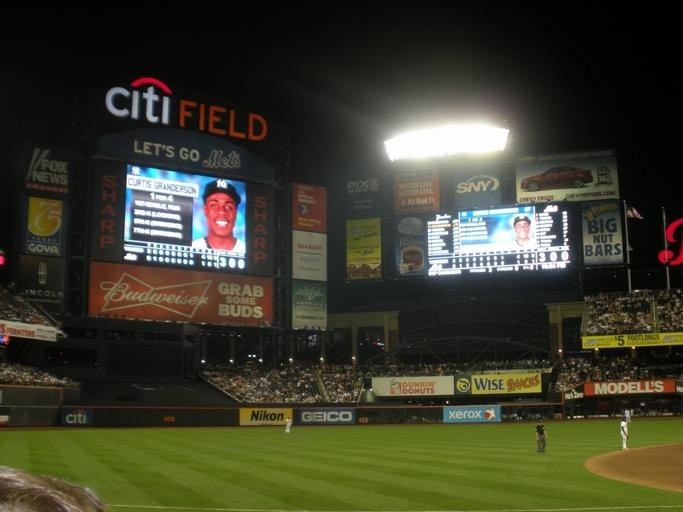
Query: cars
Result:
<box><xmin>520</xmin><ymin>166</ymin><xmax>592</xmax><ymax>192</ymax></box>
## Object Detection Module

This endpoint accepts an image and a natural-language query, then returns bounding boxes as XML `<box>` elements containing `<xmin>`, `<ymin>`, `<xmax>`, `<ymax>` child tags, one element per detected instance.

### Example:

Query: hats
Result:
<box><xmin>514</xmin><ymin>216</ymin><xmax>530</xmax><ymax>226</ymax></box>
<box><xmin>204</xmin><ymin>181</ymin><xmax>241</xmax><ymax>204</ymax></box>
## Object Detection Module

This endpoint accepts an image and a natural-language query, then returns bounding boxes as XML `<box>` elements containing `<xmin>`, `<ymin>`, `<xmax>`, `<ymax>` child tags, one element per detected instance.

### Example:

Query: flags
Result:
<box><xmin>626</xmin><ymin>204</ymin><xmax>644</xmax><ymax>221</ymax></box>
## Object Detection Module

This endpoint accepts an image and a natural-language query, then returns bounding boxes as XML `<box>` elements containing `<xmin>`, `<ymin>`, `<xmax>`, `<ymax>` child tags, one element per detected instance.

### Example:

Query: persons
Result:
<box><xmin>534</xmin><ymin>417</ymin><xmax>548</xmax><ymax>453</ymax></box>
<box><xmin>0</xmin><ymin>360</ymin><xmax>68</xmax><ymax>387</ymax></box>
<box><xmin>192</xmin><ymin>179</ymin><xmax>246</xmax><ymax>253</ymax></box>
<box><xmin>208</xmin><ymin>355</ymin><xmax>683</xmax><ymax>403</ymax></box>
<box><xmin>620</xmin><ymin>415</ymin><xmax>630</xmax><ymax>450</ymax></box>
<box><xmin>583</xmin><ymin>287</ymin><xmax>683</xmax><ymax>335</ymax></box>
<box><xmin>624</xmin><ymin>407</ymin><xmax>631</xmax><ymax>423</ymax></box>
<box><xmin>507</xmin><ymin>216</ymin><xmax>535</xmax><ymax>248</ymax></box>
<box><xmin>0</xmin><ymin>280</ymin><xmax>56</xmax><ymax>327</ymax></box>
<box><xmin>285</xmin><ymin>416</ymin><xmax>292</xmax><ymax>433</ymax></box>
